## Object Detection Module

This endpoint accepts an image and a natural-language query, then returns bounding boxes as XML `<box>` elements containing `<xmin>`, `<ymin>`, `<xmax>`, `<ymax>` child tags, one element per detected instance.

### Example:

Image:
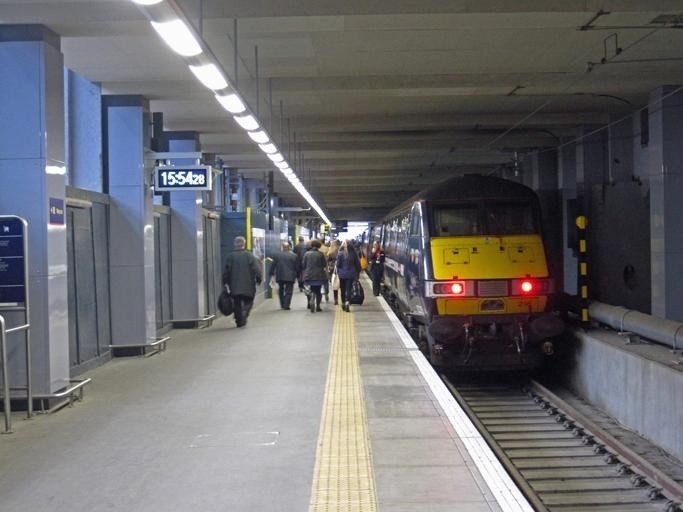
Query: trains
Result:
<box><xmin>355</xmin><ymin>173</ymin><xmax>564</xmax><ymax>386</ymax></box>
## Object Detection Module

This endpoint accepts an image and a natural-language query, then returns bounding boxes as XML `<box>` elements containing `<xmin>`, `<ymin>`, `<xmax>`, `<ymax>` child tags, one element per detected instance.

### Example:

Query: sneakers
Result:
<box><xmin>307</xmin><ymin>296</ymin><xmax>349</xmax><ymax>313</ymax></box>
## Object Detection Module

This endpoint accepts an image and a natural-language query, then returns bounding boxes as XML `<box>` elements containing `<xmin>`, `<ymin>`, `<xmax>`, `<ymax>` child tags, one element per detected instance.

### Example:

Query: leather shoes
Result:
<box><xmin>236</xmin><ymin>320</ymin><xmax>246</xmax><ymax>328</ymax></box>
<box><xmin>280</xmin><ymin>305</ymin><xmax>291</xmax><ymax>310</ymax></box>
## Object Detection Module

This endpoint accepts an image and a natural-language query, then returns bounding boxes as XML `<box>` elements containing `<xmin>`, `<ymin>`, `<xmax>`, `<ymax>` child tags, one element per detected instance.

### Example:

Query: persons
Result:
<box><xmin>270</xmin><ymin>236</ymin><xmax>365</xmax><ymax>312</ymax></box>
<box><xmin>222</xmin><ymin>236</ymin><xmax>261</xmax><ymax>327</ymax></box>
<box><xmin>369</xmin><ymin>240</ymin><xmax>385</xmax><ymax>296</ymax></box>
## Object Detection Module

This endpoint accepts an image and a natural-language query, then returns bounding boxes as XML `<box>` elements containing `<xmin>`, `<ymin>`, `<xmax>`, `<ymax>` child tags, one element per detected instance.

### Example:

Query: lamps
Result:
<box><xmin>131</xmin><ymin>0</ymin><xmax>336</xmax><ymax>227</ymax></box>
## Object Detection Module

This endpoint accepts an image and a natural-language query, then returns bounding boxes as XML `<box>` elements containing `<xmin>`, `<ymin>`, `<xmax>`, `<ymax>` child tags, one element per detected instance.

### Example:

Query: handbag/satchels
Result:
<box><xmin>331</xmin><ymin>273</ymin><xmax>340</xmax><ymax>290</ymax></box>
<box><xmin>359</xmin><ymin>254</ymin><xmax>368</xmax><ymax>269</ymax></box>
<box><xmin>217</xmin><ymin>291</ymin><xmax>234</xmax><ymax>316</ymax></box>
<box><xmin>265</xmin><ymin>285</ymin><xmax>272</xmax><ymax>298</ymax></box>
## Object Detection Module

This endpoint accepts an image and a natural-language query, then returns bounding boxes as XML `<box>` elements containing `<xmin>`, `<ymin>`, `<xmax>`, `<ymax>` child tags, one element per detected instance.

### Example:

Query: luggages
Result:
<box><xmin>350</xmin><ymin>280</ymin><xmax>364</xmax><ymax>306</ymax></box>
